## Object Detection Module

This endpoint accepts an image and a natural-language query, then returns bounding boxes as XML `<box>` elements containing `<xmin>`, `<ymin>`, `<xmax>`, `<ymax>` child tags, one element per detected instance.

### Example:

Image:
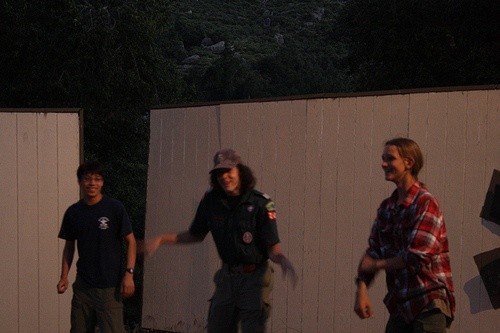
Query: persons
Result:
<box><xmin>57</xmin><ymin>161</ymin><xmax>137</xmax><ymax>333</ymax></box>
<box><xmin>353</xmin><ymin>138</ymin><xmax>456</xmax><ymax>333</ymax></box>
<box><xmin>141</xmin><ymin>150</ymin><xmax>298</xmax><ymax>333</ymax></box>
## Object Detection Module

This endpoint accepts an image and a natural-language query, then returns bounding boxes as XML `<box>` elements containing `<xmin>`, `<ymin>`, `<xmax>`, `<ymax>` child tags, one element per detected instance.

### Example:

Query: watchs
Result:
<box><xmin>125</xmin><ymin>268</ymin><xmax>135</xmax><ymax>274</ymax></box>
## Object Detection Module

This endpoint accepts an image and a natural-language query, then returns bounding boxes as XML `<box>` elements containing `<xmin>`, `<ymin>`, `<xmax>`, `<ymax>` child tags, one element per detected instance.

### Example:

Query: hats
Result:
<box><xmin>209</xmin><ymin>149</ymin><xmax>240</xmax><ymax>174</ymax></box>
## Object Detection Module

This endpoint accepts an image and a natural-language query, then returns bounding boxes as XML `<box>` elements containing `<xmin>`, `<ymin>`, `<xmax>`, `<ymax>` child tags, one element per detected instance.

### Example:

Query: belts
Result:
<box><xmin>222</xmin><ymin>261</ymin><xmax>269</xmax><ymax>272</ymax></box>
